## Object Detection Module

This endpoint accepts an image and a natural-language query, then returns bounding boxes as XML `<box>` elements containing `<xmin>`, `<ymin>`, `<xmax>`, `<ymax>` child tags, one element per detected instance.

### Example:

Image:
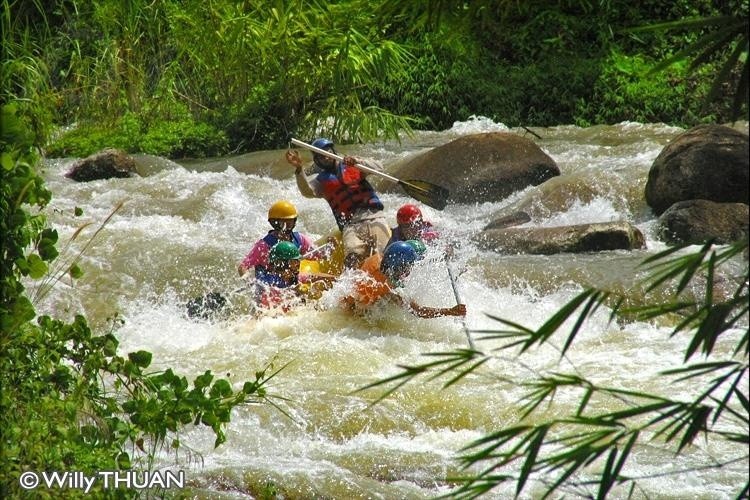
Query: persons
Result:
<box><xmin>238</xmin><ymin>139</ymin><xmax>466</xmax><ymax>319</ymax></box>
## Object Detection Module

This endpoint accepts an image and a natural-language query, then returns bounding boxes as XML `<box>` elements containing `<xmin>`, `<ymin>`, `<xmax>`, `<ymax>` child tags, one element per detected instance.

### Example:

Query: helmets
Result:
<box><xmin>381</xmin><ymin>240</ymin><xmax>426</xmax><ymax>271</ymax></box>
<box><xmin>310</xmin><ymin>138</ymin><xmax>335</xmax><ymax>160</ymax></box>
<box><xmin>269</xmin><ymin>240</ymin><xmax>303</xmax><ymax>264</ymax></box>
<box><xmin>396</xmin><ymin>205</ymin><xmax>423</xmax><ymax>225</ymax></box>
<box><xmin>267</xmin><ymin>200</ymin><xmax>298</xmax><ymax>222</ymax></box>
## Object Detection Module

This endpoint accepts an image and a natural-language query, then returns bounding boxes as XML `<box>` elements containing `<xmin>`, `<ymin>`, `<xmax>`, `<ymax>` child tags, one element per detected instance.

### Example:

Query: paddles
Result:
<box><xmin>443</xmin><ymin>212</ymin><xmax>531</xmax><ymax>236</ymax></box>
<box><xmin>187</xmin><ymin>244</ymin><xmax>331</xmax><ymax>320</ymax></box>
<box><xmin>291</xmin><ymin>138</ymin><xmax>449</xmax><ymax>211</ymax></box>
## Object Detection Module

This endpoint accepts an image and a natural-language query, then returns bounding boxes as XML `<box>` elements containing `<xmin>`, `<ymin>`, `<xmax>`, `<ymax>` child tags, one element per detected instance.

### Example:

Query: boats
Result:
<box><xmin>231</xmin><ymin>225</ymin><xmax>393</xmax><ymax>333</ymax></box>
